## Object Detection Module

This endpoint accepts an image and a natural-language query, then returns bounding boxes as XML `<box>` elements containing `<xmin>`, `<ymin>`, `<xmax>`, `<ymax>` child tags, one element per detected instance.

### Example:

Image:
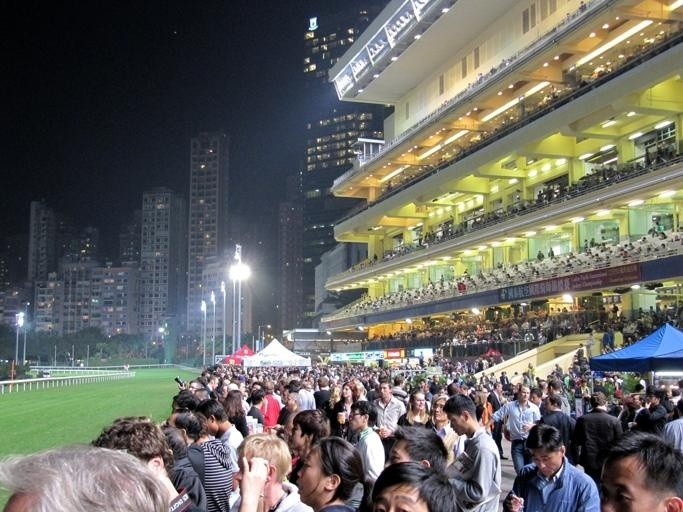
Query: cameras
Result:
<box><xmin>175</xmin><ymin>375</ymin><xmax>186</xmax><ymax>388</ymax></box>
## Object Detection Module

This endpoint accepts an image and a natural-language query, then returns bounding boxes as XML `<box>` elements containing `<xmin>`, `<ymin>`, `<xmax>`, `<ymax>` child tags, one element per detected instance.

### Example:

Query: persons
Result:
<box><xmin>1</xmin><ymin>288</ymin><xmax>683</xmax><ymax>511</ymax></box>
<box><xmin>323</xmin><ymin>1</ymin><xmax>683</xmax><ymax>315</ymax></box>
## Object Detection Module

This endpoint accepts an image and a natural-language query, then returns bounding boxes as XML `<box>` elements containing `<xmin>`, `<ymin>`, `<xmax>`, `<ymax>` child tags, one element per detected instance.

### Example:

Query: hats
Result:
<box><xmin>227</xmin><ymin>383</ymin><xmax>240</xmax><ymax>394</ymax></box>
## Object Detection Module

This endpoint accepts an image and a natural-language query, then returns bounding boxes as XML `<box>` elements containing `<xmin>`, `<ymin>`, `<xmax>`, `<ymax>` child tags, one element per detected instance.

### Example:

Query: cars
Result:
<box><xmin>42</xmin><ymin>371</ymin><xmax>50</xmax><ymax>378</ymax></box>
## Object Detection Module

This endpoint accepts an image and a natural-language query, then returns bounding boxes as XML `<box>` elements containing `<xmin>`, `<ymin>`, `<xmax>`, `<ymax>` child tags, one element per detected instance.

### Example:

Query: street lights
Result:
<box><xmin>210</xmin><ymin>290</ymin><xmax>216</xmax><ymax>366</ymax></box>
<box><xmin>200</xmin><ymin>300</ymin><xmax>206</xmax><ymax>367</ymax></box>
<box><xmin>258</xmin><ymin>324</ymin><xmax>273</xmax><ymax>352</ymax></box>
<box><xmin>221</xmin><ymin>281</ymin><xmax>226</xmax><ymax>355</ymax></box>
<box><xmin>23</xmin><ymin>302</ymin><xmax>31</xmax><ymax>367</ymax></box>
<box><xmin>16</xmin><ymin>312</ymin><xmax>25</xmax><ymax>366</ymax></box>
<box><xmin>227</xmin><ymin>244</ymin><xmax>251</xmax><ymax>354</ymax></box>
